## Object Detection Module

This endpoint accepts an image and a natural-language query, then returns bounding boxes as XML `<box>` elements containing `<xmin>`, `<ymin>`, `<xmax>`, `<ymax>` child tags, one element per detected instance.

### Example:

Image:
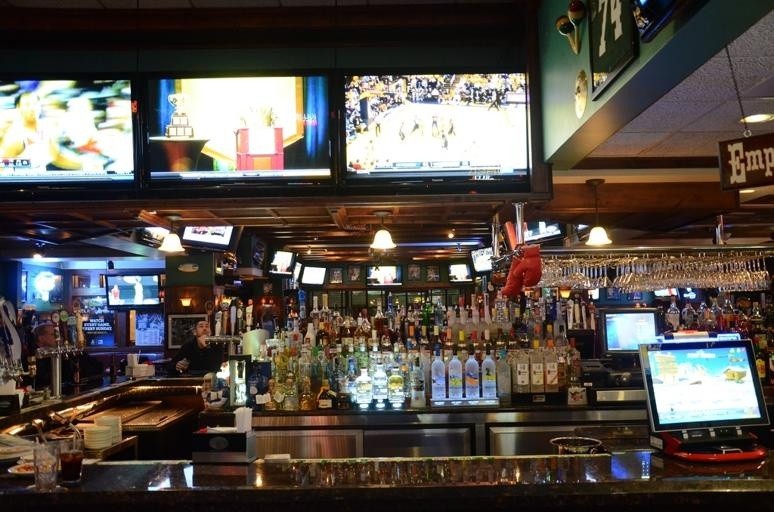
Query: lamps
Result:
<box><xmin>179</xmin><ymin>297</ymin><xmax>193</xmax><ymax>311</ymax></box>
<box><xmin>584</xmin><ymin>179</ymin><xmax>615</xmax><ymax>247</ymax></box>
<box><xmin>369</xmin><ymin>210</ymin><xmax>399</xmax><ymax>251</ymax></box>
<box><xmin>158</xmin><ymin>215</ymin><xmax>186</xmax><ymax>253</ymax></box>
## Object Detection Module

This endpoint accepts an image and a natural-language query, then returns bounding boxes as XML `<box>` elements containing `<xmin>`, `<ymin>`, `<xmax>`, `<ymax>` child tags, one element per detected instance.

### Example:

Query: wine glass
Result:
<box><xmin>615</xmin><ymin>253</ymin><xmax>716</xmax><ymax>294</ymax></box>
<box><xmin>718</xmin><ymin>251</ymin><xmax>771</xmax><ymax>292</ymax></box>
<box><xmin>535</xmin><ymin>254</ymin><xmax>613</xmax><ymax>288</ymax></box>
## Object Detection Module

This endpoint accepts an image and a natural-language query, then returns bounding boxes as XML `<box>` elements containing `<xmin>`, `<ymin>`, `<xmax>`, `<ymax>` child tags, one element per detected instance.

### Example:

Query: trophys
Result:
<box><xmin>162</xmin><ymin>93</ymin><xmax>196</xmax><ymax>139</ymax></box>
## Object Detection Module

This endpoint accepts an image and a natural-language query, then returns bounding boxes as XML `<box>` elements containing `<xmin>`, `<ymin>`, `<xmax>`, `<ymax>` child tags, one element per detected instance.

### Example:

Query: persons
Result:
<box><xmin>0</xmin><ymin>90</ymin><xmax>135</xmax><ymax>174</ymax></box>
<box><xmin>165</xmin><ymin>318</ymin><xmax>225</xmax><ymax>375</ymax></box>
<box><xmin>354</xmin><ymin>307</ymin><xmax>371</xmax><ymax>322</ymax></box>
<box><xmin>17</xmin><ymin>323</ymin><xmax>76</xmax><ymax>396</ymax></box>
<box><xmin>345</xmin><ymin>73</ymin><xmax>527</xmax><ymax>168</ymax></box>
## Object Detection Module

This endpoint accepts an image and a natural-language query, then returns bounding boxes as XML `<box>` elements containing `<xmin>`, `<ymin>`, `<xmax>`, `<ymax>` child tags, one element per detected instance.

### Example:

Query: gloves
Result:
<box><xmin>513</xmin><ymin>244</ymin><xmax>542</xmax><ymax>286</ymax></box>
<box><xmin>502</xmin><ymin>256</ymin><xmax>524</xmax><ymax>297</ymax></box>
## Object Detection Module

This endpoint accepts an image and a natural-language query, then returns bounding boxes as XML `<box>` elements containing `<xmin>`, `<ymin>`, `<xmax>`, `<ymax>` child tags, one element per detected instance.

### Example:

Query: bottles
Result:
<box><xmin>209</xmin><ymin>290</ymin><xmax>331</xmax><ymax>336</ymax></box>
<box><xmin>247</xmin><ymin>309</ymin><xmax>374</xmax><ymax>411</ymax></box>
<box><xmin>496</xmin><ymin>288</ymin><xmax>596</xmax><ymax>397</ymax></box>
<box><xmin>275</xmin><ymin>459</ymin><xmax>587</xmax><ymax>486</ymax></box>
<box><xmin>664</xmin><ymin>295</ymin><xmax>763</xmax><ymax>330</ymax></box>
<box><xmin>753</xmin><ymin>335</ymin><xmax>774</xmax><ymax>386</ymax></box>
<box><xmin>374</xmin><ymin>290</ymin><xmax>495</xmax><ymax>408</ymax></box>
<box><xmin>51</xmin><ymin>309</ymin><xmax>69</xmax><ymax>344</ymax></box>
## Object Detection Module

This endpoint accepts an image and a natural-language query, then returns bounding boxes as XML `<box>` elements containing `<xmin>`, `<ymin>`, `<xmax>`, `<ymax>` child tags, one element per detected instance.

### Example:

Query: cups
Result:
<box><xmin>33</xmin><ymin>445</ymin><xmax>60</xmax><ymax>491</ymax></box>
<box><xmin>58</xmin><ymin>439</ymin><xmax>84</xmax><ymax>483</ymax></box>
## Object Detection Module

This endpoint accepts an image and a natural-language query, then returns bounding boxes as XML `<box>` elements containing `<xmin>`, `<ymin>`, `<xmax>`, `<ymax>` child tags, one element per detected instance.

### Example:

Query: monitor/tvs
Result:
<box><xmin>105</xmin><ymin>273</ymin><xmax>161</xmax><ymax>310</ymax></box>
<box><xmin>338</xmin><ymin>65</ymin><xmax>532</xmax><ymax>194</ymax></box>
<box><xmin>269</xmin><ymin>250</ymin><xmax>326</xmax><ymax>289</ymax></box>
<box><xmin>448</xmin><ymin>221</ymin><xmax>589</xmax><ymax>284</ymax></box>
<box><xmin>139</xmin><ymin>68</ymin><xmax>339</xmax><ymax>198</ymax></box>
<box><xmin>0</xmin><ymin>71</ymin><xmax>140</xmax><ymax>201</ymax></box>
<box><xmin>366</xmin><ymin>264</ymin><xmax>402</xmax><ymax>287</ymax></box>
<box><xmin>598</xmin><ymin>305</ymin><xmax>658</xmax><ymax>354</ymax></box>
<box><xmin>639</xmin><ymin>337</ymin><xmax>771</xmax><ymax>454</ymax></box>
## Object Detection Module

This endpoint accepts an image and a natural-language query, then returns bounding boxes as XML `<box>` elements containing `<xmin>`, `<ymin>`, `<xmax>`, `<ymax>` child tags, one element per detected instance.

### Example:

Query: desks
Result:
<box><xmin>80</xmin><ymin>400</ymin><xmax>196</xmax><ymax>433</ymax></box>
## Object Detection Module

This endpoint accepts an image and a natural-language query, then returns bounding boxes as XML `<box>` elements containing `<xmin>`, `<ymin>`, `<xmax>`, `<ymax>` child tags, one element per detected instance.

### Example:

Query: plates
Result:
<box><xmin>7</xmin><ymin>461</ymin><xmax>33</xmax><ymax>477</ymax></box>
<box><xmin>94</xmin><ymin>416</ymin><xmax>123</xmax><ymax>444</ymax></box>
<box><xmin>83</xmin><ymin>427</ymin><xmax>112</xmax><ymax>449</ymax></box>
<box><xmin>551</xmin><ymin>436</ymin><xmax>602</xmax><ymax>453</ymax></box>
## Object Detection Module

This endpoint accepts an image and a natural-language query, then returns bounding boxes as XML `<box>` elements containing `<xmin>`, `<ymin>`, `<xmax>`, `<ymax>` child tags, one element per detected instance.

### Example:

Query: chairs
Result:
<box><xmin>36</xmin><ymin>412</ymin><xmax>138</xmax><ymax>462</ymax></box>
